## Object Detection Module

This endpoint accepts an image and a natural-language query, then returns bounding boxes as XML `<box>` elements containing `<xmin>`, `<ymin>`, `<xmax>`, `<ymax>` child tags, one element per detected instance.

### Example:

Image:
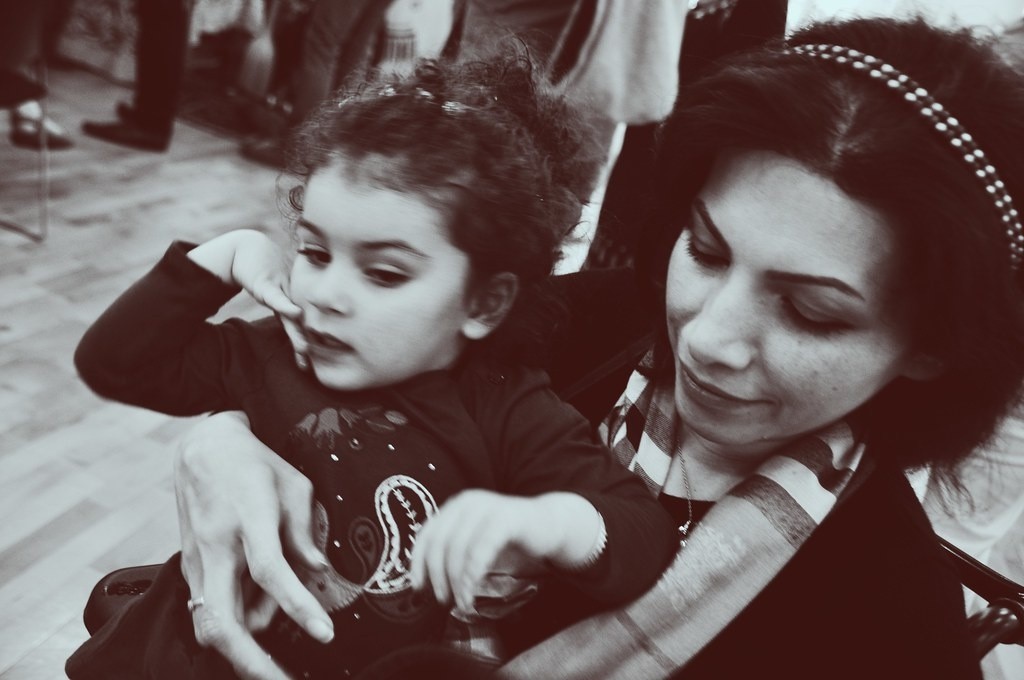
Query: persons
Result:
<box><xmin>0</xmin><ymin>0</ymin><xmax>789</xmax><ymax>170</ymax></box>
<box><xmin>170</xmin><ymin>14</ymin><xmax>1024</xmax><ymax>679</ymax></box>
<box><xmin>65</xmin><ymin>30</ymin><xmax>680</xmax><ymax>680</ymax></box>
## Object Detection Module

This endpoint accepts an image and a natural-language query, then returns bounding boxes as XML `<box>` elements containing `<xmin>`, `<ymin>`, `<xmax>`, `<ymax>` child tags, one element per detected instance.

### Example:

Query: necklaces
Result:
<box><xmin>675</xmin><ymin>424</ymin><xmax>696</xmax><ymax>549</ymax></box>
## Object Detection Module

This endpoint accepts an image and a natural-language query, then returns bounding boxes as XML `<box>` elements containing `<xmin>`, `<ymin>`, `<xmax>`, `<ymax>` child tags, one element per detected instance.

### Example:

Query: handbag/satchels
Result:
<box><xmin>546</xmin><ymin>0</ymin><xmax>693</xmax><ymax>123</ymax></box>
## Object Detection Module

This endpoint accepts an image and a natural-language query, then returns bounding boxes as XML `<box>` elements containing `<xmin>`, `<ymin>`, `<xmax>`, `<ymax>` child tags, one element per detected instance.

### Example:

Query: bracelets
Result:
<box><xmin>560</xmin><ymin>510</ymin><xmax>608</xmax><ymax>574</ymax></box>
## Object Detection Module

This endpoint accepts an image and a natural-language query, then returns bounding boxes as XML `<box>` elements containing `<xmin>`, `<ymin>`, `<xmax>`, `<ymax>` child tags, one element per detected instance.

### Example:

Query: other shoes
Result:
<box><xmin>115</xmin><ymin>98</ymin><xmax>132</xmax><ymax>122</ymax></box>
<box><xmin>241</xmin><ymin>136</ymin><xmax>289</xmax><ymax>166</ymax></box>
<box><xmin>81</xmin><ymin>118</ymin><xmax>173</xmax><ymax>152</ymax></box>
<box><xmin>10</xmin><ymin>105</ymin><xmax>74</xmax><ymax>150</ymax></box>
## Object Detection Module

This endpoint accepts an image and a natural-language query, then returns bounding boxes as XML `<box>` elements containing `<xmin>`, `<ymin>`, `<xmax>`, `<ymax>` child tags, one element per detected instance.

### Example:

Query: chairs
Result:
<box><xmin>0</xmin><ymin>0</ymin><xmax>77</xmax><ymax>242</ymax></box>
<box><xmin>82</xmin><ymin>528</ymin><xmax>1024</xmax><ymax>680</ymax></box>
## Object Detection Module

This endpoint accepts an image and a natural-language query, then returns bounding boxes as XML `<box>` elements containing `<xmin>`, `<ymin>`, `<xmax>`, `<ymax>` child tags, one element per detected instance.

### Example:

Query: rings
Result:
<box><xmin>187</xmin><ymin>596</ymin><xmax>204</xmax><ymax>612</ymax></box>
